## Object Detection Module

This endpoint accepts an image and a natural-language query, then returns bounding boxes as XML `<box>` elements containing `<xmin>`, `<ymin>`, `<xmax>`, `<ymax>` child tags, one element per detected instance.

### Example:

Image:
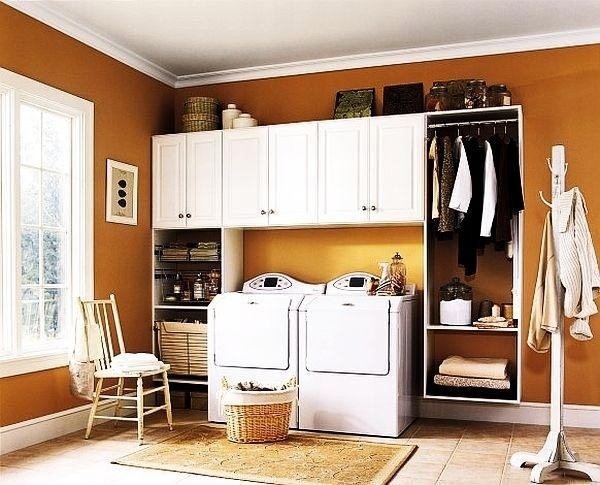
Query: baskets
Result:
<box><xmin>180</xmin><ymin>96</ymin><xmax>219</xmax><ymax>130</ymax></box>
<box><xmin>224</xmin><ymin>387</ymin><xmax>299</xmax><ymax>444</ymax></box>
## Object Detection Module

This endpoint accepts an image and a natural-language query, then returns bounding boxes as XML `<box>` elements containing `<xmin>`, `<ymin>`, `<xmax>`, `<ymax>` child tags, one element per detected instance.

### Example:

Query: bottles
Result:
<box><xmin>388</xmin><ymin>251</ymin><xmax>408</xmax><ymax>296</ymax></box>
<box><xmin>173</xmin><ymin>269</ymin><xmax>219</xmax><ymax>300</ymax></box>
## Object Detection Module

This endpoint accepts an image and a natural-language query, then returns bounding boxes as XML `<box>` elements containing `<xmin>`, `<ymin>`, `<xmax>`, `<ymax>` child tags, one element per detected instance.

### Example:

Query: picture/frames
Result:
<box><xmin>105</xmin><ymin>157</ymin><xmax>139</xmax><ymax>226</ymax></box>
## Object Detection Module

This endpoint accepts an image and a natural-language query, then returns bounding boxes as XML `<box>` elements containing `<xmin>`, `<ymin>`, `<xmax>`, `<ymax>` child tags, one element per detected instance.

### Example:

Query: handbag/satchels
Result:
<box><xmin>66</xmin><ymin>360</ymin><xmax>95</xmax><ymax>402</ymax></box>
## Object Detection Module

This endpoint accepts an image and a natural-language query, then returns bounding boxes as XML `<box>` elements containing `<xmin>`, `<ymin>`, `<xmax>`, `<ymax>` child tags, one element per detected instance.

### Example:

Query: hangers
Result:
<box><xmin>433</xmin><ymin>121</ymin><xmax>509</xmax><ymax>142</ymax></box>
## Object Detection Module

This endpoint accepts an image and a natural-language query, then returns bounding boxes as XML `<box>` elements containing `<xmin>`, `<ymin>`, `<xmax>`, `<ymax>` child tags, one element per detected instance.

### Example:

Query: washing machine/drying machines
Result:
<box><xmin>298</xmin><ymin>273</ymin><xmax>421</xmax><ymax>439</ymax></box>
<box><xmin>204</xmin><ymin>271</ymin><xmax>327</xmax><ymax>432</ymax></box>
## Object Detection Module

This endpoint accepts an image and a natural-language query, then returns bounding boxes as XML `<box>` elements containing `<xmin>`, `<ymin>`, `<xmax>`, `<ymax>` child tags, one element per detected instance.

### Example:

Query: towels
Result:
<box><xmin>108</xmin><ymin>351</ymin><xmax>161</xmax><ymax>374</ymax></box>
<box><xmin>434</xmin><ymin>355</ymin><xmax>512</xmax><ymax>390</ymax></box>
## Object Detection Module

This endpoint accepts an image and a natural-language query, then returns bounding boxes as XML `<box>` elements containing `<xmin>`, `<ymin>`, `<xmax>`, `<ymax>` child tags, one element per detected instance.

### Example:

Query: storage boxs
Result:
<box><xmin>158</xmin><ymin>390</ymin><xmax>207</xmax><ymax>411</ymax></box>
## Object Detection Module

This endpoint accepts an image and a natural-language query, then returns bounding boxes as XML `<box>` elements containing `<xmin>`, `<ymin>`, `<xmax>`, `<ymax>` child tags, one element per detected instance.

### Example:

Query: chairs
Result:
<box><xmin>77</xmin><ymin>294</ymin><xmax>173</xmax><ymax>446</ymax></box>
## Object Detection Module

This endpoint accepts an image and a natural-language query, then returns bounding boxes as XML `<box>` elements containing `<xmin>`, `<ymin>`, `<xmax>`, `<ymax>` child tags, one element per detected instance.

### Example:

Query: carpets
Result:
<box><xmin>109</xmin><ymin>418</ymin><xmax>417</xmax><ymax>485</ymax></box>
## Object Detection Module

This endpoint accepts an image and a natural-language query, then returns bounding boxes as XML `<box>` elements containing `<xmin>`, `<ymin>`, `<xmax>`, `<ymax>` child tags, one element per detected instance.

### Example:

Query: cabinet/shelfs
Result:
<box><xmin>315</xmin><ymin>114</ymin><xmax>423</xmax><ymax>222</ymax></box>
<box><xmin>149</xmin><ymin>133</ymin><xmax>222</xmax><ymax>228</ymax></box>
<box><xmin>148</xmin><ymin>227</ymin><xmax>245</xmax><ymax>385</ymax></box>
<box><xmin>224</xmin><ymin>122</ymin><xmax>315</xmax><ymax>225</ymax></box>
<box><xmin>424</xmin><ymin>108</ymin><xmax>526</xmax><ymax>406</ymax></box>
